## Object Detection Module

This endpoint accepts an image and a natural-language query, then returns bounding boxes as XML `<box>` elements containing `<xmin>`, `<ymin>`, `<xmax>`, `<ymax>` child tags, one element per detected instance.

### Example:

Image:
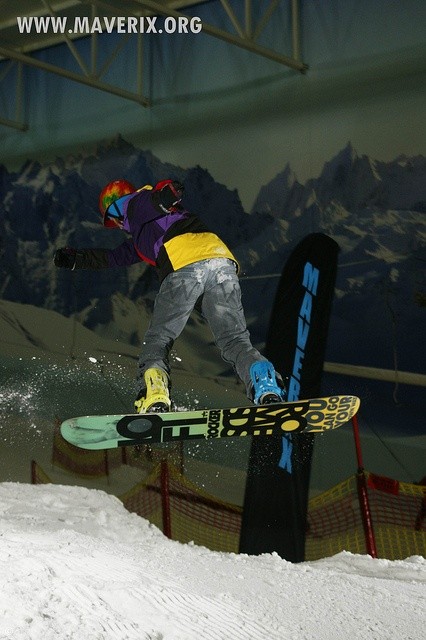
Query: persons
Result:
<box><xmin>52</xmin><ymin>180</ymin><xmax>287</xmax><ymax>416</ymax></box>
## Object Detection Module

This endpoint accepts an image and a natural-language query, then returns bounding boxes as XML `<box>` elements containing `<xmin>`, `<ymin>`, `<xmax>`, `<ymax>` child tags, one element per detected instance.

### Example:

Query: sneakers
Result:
<box><xmin>134</xmin><ymin>367</ymin><xmax>177</xmax><ymax>413</ymax></box>
<box><xmin>251</xmin><ymin>361</ymin><xmax>287</xmax><ymax>406</ymax></box>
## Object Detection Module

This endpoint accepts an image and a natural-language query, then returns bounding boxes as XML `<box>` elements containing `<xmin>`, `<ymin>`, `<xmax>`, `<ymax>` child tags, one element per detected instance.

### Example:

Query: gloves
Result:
<box><xmin>54</xmin><ymin>248</ymin><xmax>74</xmax><ymax>269</ymax></box>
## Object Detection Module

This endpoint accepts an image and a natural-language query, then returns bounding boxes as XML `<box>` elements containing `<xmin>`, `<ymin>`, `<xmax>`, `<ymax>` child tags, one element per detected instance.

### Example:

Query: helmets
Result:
<box><xmin>99</xmin><ymin>179</ymin><xmax>136</xmax><ymax>229</ymax></box>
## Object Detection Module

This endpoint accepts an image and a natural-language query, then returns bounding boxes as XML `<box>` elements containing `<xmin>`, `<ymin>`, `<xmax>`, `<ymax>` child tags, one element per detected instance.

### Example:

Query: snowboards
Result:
<box><xmin>60</xmin><ymin>395</ymin><xmax>361</xmax><ymax>449</ymax></box>
